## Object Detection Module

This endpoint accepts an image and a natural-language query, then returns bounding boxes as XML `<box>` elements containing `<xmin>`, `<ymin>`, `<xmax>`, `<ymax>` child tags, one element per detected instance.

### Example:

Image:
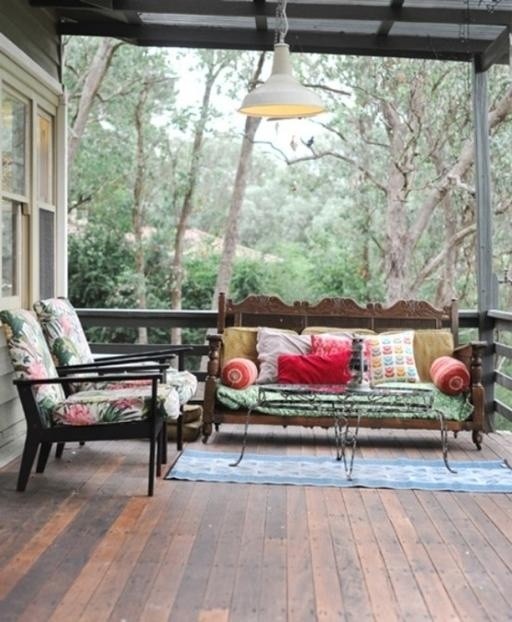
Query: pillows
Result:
<box><xmin>249</xmin><ymin>326</ymin><xmax>425</xmax><ymax>387</ymax></box>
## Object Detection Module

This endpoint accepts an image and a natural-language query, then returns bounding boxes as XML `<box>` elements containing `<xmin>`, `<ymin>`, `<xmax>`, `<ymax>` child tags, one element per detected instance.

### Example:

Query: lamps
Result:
<box><xmin>235</xmin><ymin>0</ymin><xmax>326</xmax><ymax>120</ymax></box>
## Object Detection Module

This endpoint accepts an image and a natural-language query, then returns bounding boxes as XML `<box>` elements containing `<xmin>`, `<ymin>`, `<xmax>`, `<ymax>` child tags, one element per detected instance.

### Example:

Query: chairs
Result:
<box><xmin>1</xmin><ymin>305</ymin><xmax>181</xmax><ymax>496</ymax></box>
<box><xmin>33</xmin><ymin>294</ymin><xmax>198</xmax><ymax>465</ymax></box>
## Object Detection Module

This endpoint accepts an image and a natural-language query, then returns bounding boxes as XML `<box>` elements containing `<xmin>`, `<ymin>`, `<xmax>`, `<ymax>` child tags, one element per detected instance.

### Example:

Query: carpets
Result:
<box><xmin>163</xmin><ymin>449</ymin><xmax>512</xmax><ymax>494</ymax></box>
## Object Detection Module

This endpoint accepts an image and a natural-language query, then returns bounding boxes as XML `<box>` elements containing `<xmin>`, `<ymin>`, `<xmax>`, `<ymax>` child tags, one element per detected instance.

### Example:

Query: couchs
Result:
<box><xmin>202</xmin><ymin>293</ymin><xmax>489</xmax><ymax>452</ymax></box>
